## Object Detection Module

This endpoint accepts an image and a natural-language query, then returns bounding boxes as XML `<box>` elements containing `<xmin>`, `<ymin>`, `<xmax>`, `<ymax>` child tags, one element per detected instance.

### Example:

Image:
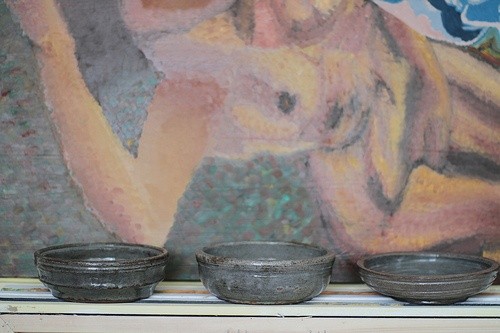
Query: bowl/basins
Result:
<box><xmin>35</xmin><ymin>242</ymin><xmax>169</xmax><ymax>306</ymax></box>
<box><xmin>197</xmin><ymin>240</ymin><xmax>334</xmax><ymax>308</ymax></box>
<box><xmin>353</xmin><ymin>251</ymin><xmax>497</xmax><ymax>304</ymax></box>
